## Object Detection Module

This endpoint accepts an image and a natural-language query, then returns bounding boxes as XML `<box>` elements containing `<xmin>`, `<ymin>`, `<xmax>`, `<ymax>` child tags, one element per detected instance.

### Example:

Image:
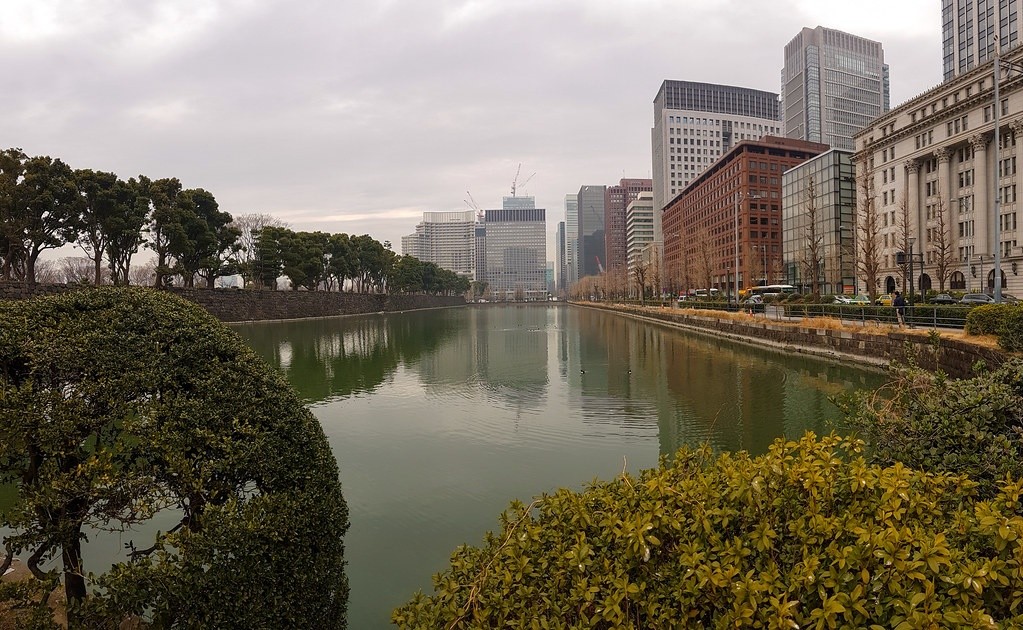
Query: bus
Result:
<box><xmin>751</xmin><ymin>284</ymin><xmax>798</xmax><ymax>304</ymax></box>
<box><xmin>695</xmin><ymin>288</ymin><xmax>719</xmax><ymax>298</ymax></box>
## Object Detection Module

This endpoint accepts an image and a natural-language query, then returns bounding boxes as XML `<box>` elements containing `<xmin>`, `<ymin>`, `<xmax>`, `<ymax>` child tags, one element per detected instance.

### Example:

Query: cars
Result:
<box><xmin>875</xmin><ymin>293</ymin><xmax>910</xmax><ymax>306</ymax></box>
<box><xmin>678</xmin><ymin>296</ymin><xmax>687</xmax><ymax>303</ymax></box>
<box><xmin>929</xmin><ymin>294</ymin><xmax>962</xmax><ymax>305</ymax></box>
<box><xmin>962</xmin><ymin>292</ymin><xmax>1023</xmax><ymax>305</ymax></box>
<box><xmin>820</xmin><ymin>293</ymin><xmax>871</xmax><ymax>306</ymax></box>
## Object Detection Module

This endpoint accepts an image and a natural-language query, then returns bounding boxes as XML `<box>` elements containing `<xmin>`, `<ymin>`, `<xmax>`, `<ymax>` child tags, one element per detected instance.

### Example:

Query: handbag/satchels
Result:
<box><xmin>899</xmin><ymin>309</ymin><xmax>907</xmax><ymax>314</ymax></box>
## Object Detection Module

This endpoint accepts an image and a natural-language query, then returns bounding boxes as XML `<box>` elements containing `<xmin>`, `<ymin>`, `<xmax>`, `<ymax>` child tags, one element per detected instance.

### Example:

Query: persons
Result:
<box><xmin>893</xmin><ymin>291</ymin><xmax>907</xmax><ymax>331</ymax></box>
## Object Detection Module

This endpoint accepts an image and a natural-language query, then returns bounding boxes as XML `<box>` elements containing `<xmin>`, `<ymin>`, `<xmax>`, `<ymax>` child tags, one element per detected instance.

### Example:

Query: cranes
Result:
<box><xmin>463</xmin><ymin>190</ymin><xmax>484</xmax><ymax>222</ymax></box>
<box><xmin>511</xmin><ymin>163</ymin><xmax>537</xmax><ymax>197</ymax></box>
